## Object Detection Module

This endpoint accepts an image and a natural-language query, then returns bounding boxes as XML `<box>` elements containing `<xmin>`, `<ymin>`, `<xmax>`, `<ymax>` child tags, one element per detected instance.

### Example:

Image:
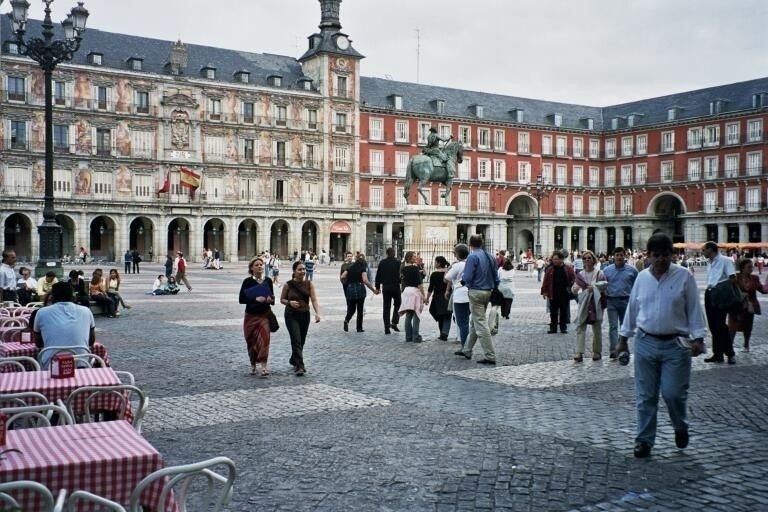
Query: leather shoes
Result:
<box><xmin>390</xmin><ymin>323</ymin><xmax>399</xmax><ymax>332</ymax></box>
<box><xmin>704</xmin><ymin>354</ymin><xmax>725</xmax><ymax>363</ymax></box>
<box><xmin>634</xmin><ymin>444</ymin><xmax>651</xmax><ymax>458</ymax></box>
<box><xmin>384</xmin><ymin>328</ymin><xmax>392</xmax><ymax>335</ymax></box>
<box><xmin>727</xmin><ymin>355</ymin><xmax>737</xmax><ymax>363</ymax></box>
<box><xmin>675</xmin><ymin>432</ymin><xmax>689</xmax><ymax>448</ymax></box>
<box><xmin>455</xmin><ymin>350</ymin><xmax>471</xmax><ymax>359</ymax></box>
<box><xmin>477</xmin><ymin>358</ymin><xmax>496</xmax><ymax>365</ymax></box>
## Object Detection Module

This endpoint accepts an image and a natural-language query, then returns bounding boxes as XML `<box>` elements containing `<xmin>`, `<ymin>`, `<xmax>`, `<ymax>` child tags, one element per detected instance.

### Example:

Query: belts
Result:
<box><xmin>639</xmin><ymin>328</ymin><xmax>669</xmax><ymax>340</ymax></box>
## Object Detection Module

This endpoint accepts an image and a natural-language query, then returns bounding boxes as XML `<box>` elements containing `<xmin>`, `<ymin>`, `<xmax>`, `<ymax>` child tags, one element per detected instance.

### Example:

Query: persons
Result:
<box><xmin>340</xmin><ymin>234</ymin><xmax>516</xmax><ymax>367</ymax></box>
<box><xmin>238</xmin><ymin>256</ymin><xmax>276</xmax><ymax>377</ymax></box>
<box><xmin>540</xmin><ymin>247</ymin><xmax>639</xmax><ymax>362</ymax></box>
<box><xmin>280</xmin><ymin>260</ymin><xmax>322</xmax><ymax>376</ymax></box>
<box><xmin>0</xmin><ymin>246</ymin><xmax>220</xmax><ymax>319</ymax></box>
<box><xmin>614</xmin><ymin>232</ymin><xmax>707</xmax><ymax>459</ymax></box>
<box><xmin>422</xmin><ymin>125</ymin><xmax>453</xmax><ymax>164</ymax></box>
<box><xmin>703</xmin><ymin>241</ymin><xmax>768</xmax><ymax>366</ymax></box>
<box><xmin>32</xmin><ymin>281</ymin><xmax>95</xmax><ymax>370</ymax></box>
<box><xmin>518</xmin><ymin>245</ymin><xmax>768</xmax><ymax>282</ymax></box>
<box><xmin>259</xmin><ymin>248</ymin><xmax>329</xmax><ymax>281</ymax></box>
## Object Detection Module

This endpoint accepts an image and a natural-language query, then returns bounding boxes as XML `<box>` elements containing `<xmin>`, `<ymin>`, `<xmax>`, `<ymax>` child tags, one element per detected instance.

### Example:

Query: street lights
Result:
<box><xmin>2</xmin><ymin>1</ymin><xmax>90</xmax><ymax>285</ymax></box>
<box><xmin>524</xmin><ymin>173</ymin><xmax>556</xmax><ymax>260</ymax></box>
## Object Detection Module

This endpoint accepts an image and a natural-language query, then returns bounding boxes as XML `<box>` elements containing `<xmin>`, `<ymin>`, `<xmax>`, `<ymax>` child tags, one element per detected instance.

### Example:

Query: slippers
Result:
<box><xmin>344</xmin><ymin>320</ymin><xmax>348</xmax><ymax>332</ymax></box>
<box><xmin>357</xmin><ymin>329</ymin><xmax>365</xmax><ymax>332</ymax></box>
<box><xmin>262</xmin><ymin>369</ymin><xmax>269</xmax><ymax>376</ymax></box>
<box><xmin>385</xmin><ymin>329</ymin><xmax>390</xmax><ymax>334</ymax></box>
<box><xmin>250</xmin><ymin>369</ymin><xmax>257</xmax><ymax>375</ymax></box>
<box><xmin>438</xmin><ymin>335</ymin><xmax>447</xmax><ymax>340</ymax></box>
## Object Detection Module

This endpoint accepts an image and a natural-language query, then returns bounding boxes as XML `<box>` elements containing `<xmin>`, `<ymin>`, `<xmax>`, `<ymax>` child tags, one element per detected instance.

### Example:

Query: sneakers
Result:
<box><xmin>610</xmin><ymin>351</ymin><xmax>618</xmax><ymax>361</ymax></box>
<box><xmin>574</xmin><ymin>353</ymin><xmax>584</xmax><ymax>361</ymax></box>
<box><xmin>547</xmin><ymin>320</ymin><xmax>571</xmax><ymax>333</ymax></box>
<box><xmin>592</xmin><ymin>353</ymin><xmax>601</xmax><ymax>361</ymax></box>
<box><xmin>295</xmin><ymin>368</ymin><xmax>304</xmax><ymax>374</ymax></box>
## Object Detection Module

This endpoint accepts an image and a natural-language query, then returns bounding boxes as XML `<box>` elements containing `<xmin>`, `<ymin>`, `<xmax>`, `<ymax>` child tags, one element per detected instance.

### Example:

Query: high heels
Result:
<box><xmin>502</xmin><ymin>316</ymin><xmax>505</xmax><ymax>318</ymax></box>
<box><xmin>506</xmin><ymin>316</ymin><xmax>509</xmax><ymax>319</ymax></box>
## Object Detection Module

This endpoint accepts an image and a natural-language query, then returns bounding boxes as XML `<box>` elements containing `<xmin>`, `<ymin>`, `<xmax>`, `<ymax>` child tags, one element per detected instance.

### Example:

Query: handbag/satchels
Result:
<box><xmin>490</xmin><ymin>288</ymin><xmax>506</xmax><ymax>306</ymax></box>
<box><xmin>269</xmin><ymin>310</ymin><xmax>280</xmax><ymax>332</ymax></box>
<box><xmin>488</xmin><ymin>310</ymin><xmax>499</xmax><ymax>335</ymax></box>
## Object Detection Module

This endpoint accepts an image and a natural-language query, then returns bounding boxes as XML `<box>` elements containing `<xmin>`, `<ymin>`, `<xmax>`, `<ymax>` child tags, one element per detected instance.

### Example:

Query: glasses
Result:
<box><xmin>582</xmin><ymin>256</ymin><xmax>592</xmax><ymax>260</ymax></box>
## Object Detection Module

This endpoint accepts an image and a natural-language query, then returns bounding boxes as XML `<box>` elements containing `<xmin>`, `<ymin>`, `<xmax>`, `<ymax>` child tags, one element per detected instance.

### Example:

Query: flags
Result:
<box><xmin>155</xmin><ymin>170</ymin><xmax>170</xmax><ymax>198</ymax></box>
<box><xmin>190</xmin><ymin>170</ymin><xmax>196</xmax><ymax>201</ymax></box>
<box><xmin>178</xmin><ymin>167</ymin><xmax>201</xmax><ymax>190</ymax></box>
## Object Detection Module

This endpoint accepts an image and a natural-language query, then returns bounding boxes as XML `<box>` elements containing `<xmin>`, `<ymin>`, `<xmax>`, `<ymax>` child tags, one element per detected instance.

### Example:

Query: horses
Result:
<box><xmin>402</xmin><ymin>139</ymin><xmax>464</xmax><ymax>206</ymax></box>
